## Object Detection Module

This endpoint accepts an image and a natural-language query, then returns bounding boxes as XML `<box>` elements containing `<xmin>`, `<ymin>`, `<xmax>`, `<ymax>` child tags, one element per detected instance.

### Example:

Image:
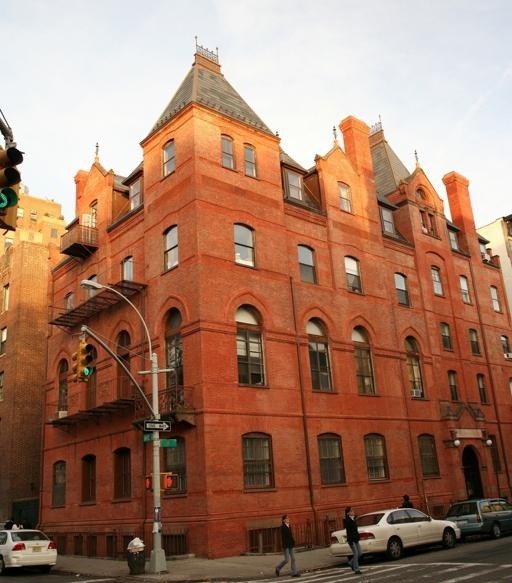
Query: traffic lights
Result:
<box><xmin>71</xmin><ymin>343</ymin><xmax>94</xmax><ymax>382</ymax></box>
<box><xmin>163</xmin><ymin>475</ymin><xmax>179</xmax><ymax>490</ymax></box>
<box><xmin>0</xmin><ymin>145</ymin><xmax>23</xmax><ymax>232</ymax></box>
<box><xmin>145</xmin><ymin>475</ymin><xmax>152</xmax><ymax>493</ymax></box>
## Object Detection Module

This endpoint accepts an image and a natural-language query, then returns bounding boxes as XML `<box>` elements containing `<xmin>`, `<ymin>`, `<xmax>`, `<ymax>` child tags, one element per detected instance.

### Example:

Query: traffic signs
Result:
<box><xmin>144</xmin><ymin>420</ymin><xmax>172</xmax><ymax>433</ymax></box>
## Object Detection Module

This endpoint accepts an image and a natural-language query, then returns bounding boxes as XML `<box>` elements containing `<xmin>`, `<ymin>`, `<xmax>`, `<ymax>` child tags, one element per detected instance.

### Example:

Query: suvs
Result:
<box><xmin>444</xmin><ymin>498</ymin><xmax>512</xmax><ymax>539</ymax></box>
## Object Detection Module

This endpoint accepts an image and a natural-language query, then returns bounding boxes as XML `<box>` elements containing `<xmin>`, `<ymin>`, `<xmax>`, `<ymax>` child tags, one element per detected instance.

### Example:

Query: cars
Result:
<box><xmin>330</xmin><ymin>509</ymin><xmax>462</xmax><ymax>564</ymax></box>
<box><xmin>0</xmin><ymin>529</ymin><xmax>58</xmax><ymax>575</ymax></box>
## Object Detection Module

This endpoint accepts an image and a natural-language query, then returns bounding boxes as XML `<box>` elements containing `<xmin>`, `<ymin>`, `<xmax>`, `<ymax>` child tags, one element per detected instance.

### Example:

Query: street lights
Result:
<box><xmin>80</xmin><ymin>279</ymin><xmax>166</xmax><ymax>572</ymax></box>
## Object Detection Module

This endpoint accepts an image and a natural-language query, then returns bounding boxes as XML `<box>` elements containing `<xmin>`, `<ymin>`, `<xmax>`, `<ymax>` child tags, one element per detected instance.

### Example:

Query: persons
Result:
<box><xmin>398</xmin><ymin>494</ymin><xmax>414</xmax><ymax>508</ymax></box>
<box><xmin>3</xmin><ymin>519</ymin><xmax>35</xmax><ymax>529</ymax></box>
<box><xmin>275</xmin><ymin>514</ymin><xmax>301</xmax><ymax>577</ymax></box>
<box><xmin>343</xmin><ymin>506</ymin><xmax>363</xmax><ymax>575</ymax></box>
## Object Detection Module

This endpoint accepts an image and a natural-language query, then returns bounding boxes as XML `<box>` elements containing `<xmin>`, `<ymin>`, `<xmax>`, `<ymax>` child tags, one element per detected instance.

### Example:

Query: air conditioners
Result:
<box><xmin>506</xmin><ymin>353</ymin><xmax>512</xmax><ymax>358</ymax></box>
<box><xmin>483</xmin><ymin>254</ymin><xmax>490</xmax><ymax>261</ymax></box>
<box><xmin>410</xmin><ymin>389</ymin><xmax>421</xmax><ymax>397</ymax></box>
<box><xmin>56</xmin><ymin>411</ymin><xmax>68</xmax><ymax>419</ymax></box>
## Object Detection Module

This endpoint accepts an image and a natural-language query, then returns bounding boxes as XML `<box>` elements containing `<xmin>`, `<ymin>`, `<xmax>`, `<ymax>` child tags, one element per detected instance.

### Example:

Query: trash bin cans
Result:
<box><xmin>126</xmin><ymin>538</ymin><xmax>147</xmax><ymax>575</ymax></box>
